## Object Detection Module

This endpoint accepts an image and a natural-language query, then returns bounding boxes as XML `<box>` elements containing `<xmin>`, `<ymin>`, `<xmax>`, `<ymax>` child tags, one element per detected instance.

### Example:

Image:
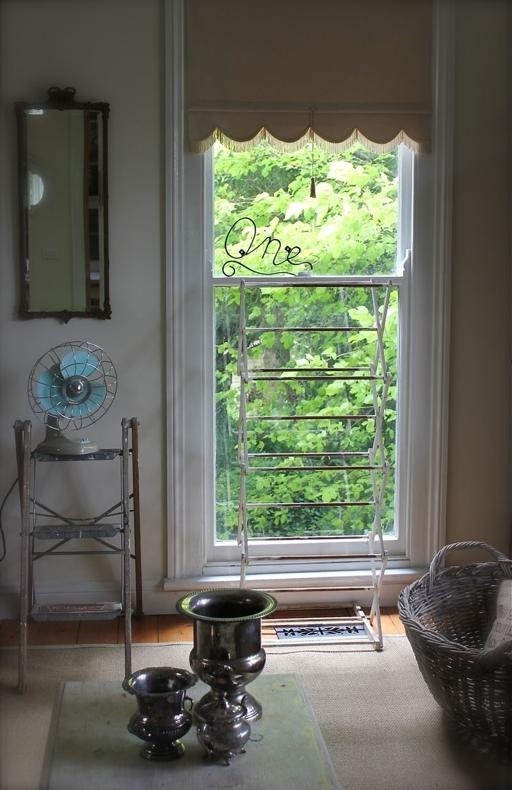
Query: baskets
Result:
<box><xmin>398</xmin><ymin>540</ymin><xmax>512</xmax><ymax>745</ymax></box>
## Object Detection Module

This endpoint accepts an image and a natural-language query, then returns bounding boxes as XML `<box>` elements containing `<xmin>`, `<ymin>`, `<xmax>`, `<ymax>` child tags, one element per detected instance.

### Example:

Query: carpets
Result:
<box><xmin>0</xmin><ymin>634</ymin><xmax>509</xmax><ymax>788</ymax></box>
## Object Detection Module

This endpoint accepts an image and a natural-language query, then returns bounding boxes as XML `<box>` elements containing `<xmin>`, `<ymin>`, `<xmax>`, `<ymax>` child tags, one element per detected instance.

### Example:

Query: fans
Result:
<box><xmin>22</xmin><ymin>337</ymin><xmax>121</xmax><ymax>459</ymax></box>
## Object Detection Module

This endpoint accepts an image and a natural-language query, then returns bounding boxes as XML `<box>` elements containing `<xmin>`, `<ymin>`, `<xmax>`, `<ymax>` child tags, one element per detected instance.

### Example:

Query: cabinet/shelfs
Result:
<box><xmin>232</xmin><ymin>276</ymin><xmax>399</xmax><ymax>655</ymax></box>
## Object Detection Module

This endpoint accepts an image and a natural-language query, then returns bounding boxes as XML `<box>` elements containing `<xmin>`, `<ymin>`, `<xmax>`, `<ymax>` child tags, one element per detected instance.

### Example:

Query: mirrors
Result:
<box><xmin>15</xmin><ymin>87</ymin><xmax>113</xmax><ymax>326</ymax></box>
